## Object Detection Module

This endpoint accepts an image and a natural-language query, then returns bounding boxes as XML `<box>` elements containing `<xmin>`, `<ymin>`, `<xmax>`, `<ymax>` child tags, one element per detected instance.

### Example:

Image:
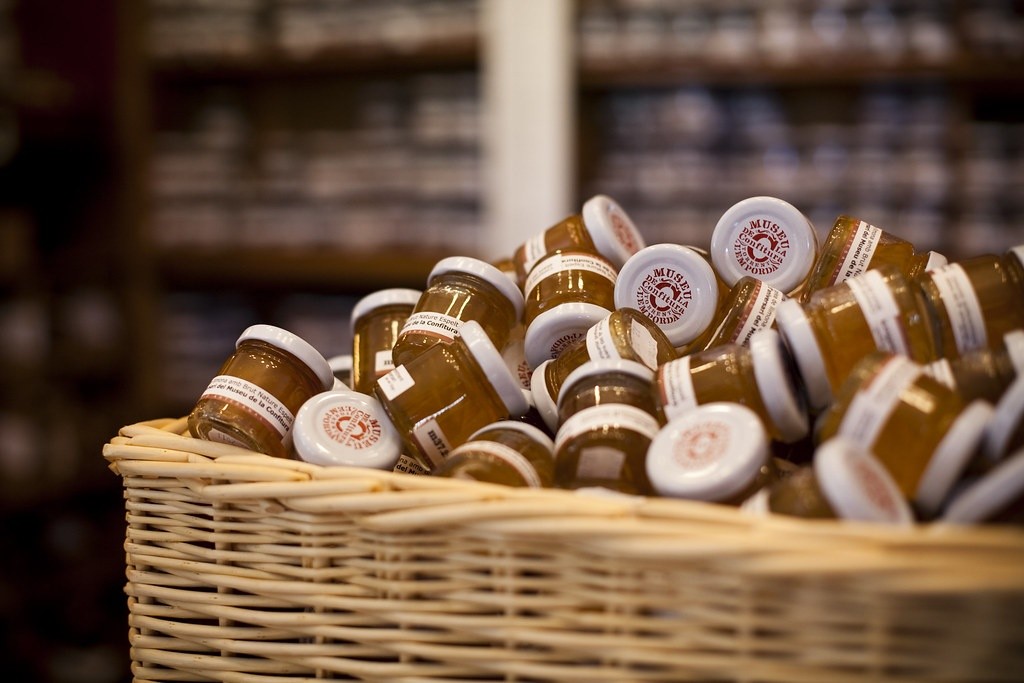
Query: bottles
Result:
<box><xmin>187</xmin><ymin>193</ymin><xmax>1024</xmax><ymax>523</ymax></box>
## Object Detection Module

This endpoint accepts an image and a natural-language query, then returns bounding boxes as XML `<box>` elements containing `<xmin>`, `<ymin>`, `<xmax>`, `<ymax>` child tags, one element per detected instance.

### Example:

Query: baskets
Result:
<box><xmin>102</xmin><ymin>411</ymin><xmax>1024</xmax><ymax>683</ymax></box>
